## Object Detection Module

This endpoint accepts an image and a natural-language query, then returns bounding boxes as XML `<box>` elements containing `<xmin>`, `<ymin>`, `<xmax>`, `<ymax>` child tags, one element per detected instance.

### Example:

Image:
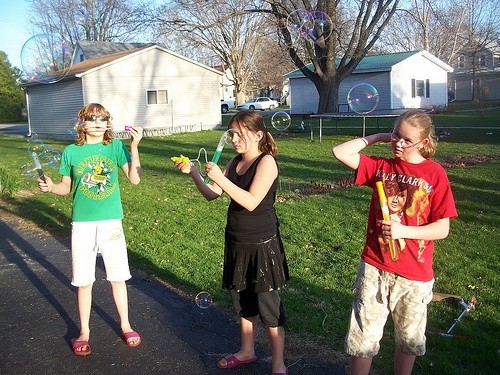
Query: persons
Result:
<box><xmin>174</xmin><ymin>111</ymin><xmax>292</xmax><ymax>375</ymax></box>
<box><xmin>332</xmin><ymin>110</ymin><xmax>458</xmax><ymax>375</ymax></box>
<box><xmin>38</xmin><ymin>103</ymin><xmax>143</xmax><ymax>356</ymax></box>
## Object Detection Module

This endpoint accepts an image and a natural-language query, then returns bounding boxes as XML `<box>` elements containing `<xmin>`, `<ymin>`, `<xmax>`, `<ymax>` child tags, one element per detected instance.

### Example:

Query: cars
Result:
<box><xmin>235</xmin><ymin>97</ymin><xmax>279</xmax><ymax>112</ymax></box>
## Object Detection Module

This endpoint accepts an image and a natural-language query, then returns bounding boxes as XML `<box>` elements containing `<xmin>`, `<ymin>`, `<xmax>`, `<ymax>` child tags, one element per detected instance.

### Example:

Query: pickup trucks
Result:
<box><xmin>221</xmin><ymin>99</ymin><xmax>235</xmax><ymax>114</ymax></box>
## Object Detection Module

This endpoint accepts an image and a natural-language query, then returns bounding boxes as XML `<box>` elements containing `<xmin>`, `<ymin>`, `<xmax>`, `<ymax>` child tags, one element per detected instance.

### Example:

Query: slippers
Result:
<box><xmin>217</xmin><ymin>353</ymin><xmax>257</xmax><ymax>369</ymax></box>
<box><xmin>123</xmin><ymin>331</ymin><xmax>141</xmax><ymax>346</ymax></box>
<box><xmin>70</xmin><ymin>337</ymin><xmax>91</xmax><ymax>356</ymax></box>
<box><xmin>272</xmin><ymin>367</ymin><xmax>289</xmax><ymax>375</ymax></box>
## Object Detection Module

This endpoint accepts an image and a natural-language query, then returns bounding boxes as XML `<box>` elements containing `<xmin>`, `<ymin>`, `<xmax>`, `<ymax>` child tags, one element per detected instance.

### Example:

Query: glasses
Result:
<box><xmin>84</xmin><ymin>115</ymin><xmax>108</xmax><ymax>122</ymax></box>
<box><xmin>389</xmin><ymin>128</ymin><xmax>424</xmax><ymax>149</ymax></box>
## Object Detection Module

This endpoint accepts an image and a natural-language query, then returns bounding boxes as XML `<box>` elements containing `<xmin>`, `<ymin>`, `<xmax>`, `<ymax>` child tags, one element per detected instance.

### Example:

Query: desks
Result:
<box><xmin>310</xmin><ymin>109</ymin><xmax>434</xmax><ymax>141</ymax></box>
